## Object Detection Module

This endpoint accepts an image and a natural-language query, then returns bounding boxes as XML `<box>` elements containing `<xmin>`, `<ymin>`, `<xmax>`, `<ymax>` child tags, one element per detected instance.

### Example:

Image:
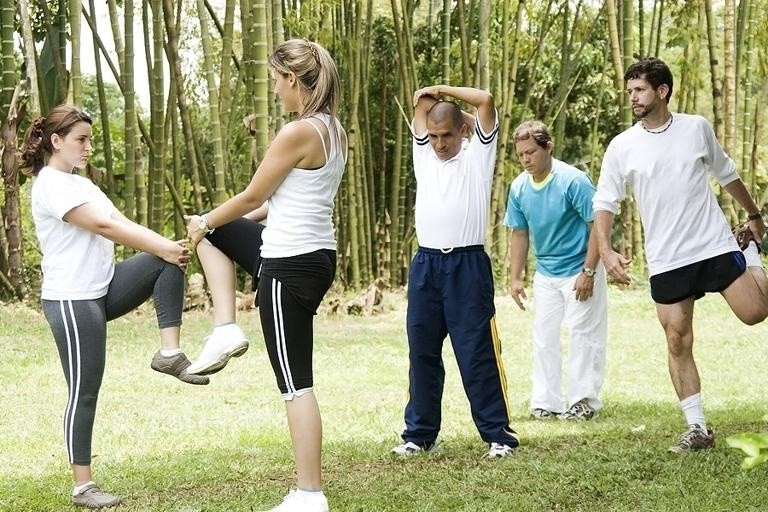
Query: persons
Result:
<box><xmin>589</xmin><ymin>56</ymin><xmax>767</xmax><ymax>457</ymax></box>
<box><xmin>502</xmin><ymin>119</ymin><xmax>608</xmax><ymax>422</ymax></box>
<box><xmin>182</xmin><ymin>38</ymin><xmax>350</xmax><ymax>512</ymax></box>
<box><xmin>389</xmin><ymin>83</ymin><xmax>521</xmax><ymax>463</ymax></box>
<box><xmin>16</xmin><ymin>104</ymin><xmax>210</xmax><ymax>508</ymax></box>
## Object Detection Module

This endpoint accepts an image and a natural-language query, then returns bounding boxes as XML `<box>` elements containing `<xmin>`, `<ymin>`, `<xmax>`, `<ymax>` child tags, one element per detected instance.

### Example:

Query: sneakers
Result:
<box><xmin>667</xmin><ymin>423</ymin><xmax>716</xmax><ymax>455</ymax></box>
<box><xmin>150</xmin><ymin>349</ymin><xmax>210</xmax><ymax>386</ymax></box>
<box><xmin>731</xmin><ymin>217</ymin><xmax>768</xmax><ymax>252</ymax></box>
<box><xmin>71</xmin><ymin>484</ymin><xmax>120</xmax><ymax>508</ymax></box>
<box><xmin>266</xmin><ymin>488</ymin><xmax>329</xmax><ymax>512</ymax></box>
<box><xmin>185</xmin><ymin>323</ymin><xmax>250</xmax><ymax>375</ymax></box>
<box><xmin>528</xmin><ymin>408</ymin><xmax>555</xmax><ymax>420</ymax></box>
<box><xmin>390</xmin><ymin>439</ymin><xmax>441</xmax><ymax>458</ymax></box>
<box><xmin>556</xmin><ymin>402</ymin><xmax>600</xmax><ymax>422</ymax></box>
<box><xmin>480</xmin><ymin>442</ymin><xmax>514</xmax><ymax>461</ymax></box>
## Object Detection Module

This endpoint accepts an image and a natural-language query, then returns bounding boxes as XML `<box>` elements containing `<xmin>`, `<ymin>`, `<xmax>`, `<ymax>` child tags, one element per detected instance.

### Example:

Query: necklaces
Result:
<box><xmin>641</xmin><ymin>111</ymin><xmax>674</xmax><ymax>135</ymax></box>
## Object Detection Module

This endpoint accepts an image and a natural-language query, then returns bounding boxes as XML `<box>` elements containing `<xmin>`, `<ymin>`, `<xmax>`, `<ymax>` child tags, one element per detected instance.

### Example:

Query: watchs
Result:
<box><xmin>197</xmin><ymin>214</ymin><xmax>216</xmax><ymax>235</ymax></box>
<box><xmin>581</xmin><ymin>267</ymin><xmax>596</xmax><ymax>277</ymax></box>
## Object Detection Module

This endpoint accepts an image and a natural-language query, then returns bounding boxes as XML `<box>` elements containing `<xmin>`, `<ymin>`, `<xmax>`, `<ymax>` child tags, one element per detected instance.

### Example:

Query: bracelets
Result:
<box><xmin>747</xmin><ymin>212</ymin><xmax>762</xmax><ymax>221</ymax></box>
